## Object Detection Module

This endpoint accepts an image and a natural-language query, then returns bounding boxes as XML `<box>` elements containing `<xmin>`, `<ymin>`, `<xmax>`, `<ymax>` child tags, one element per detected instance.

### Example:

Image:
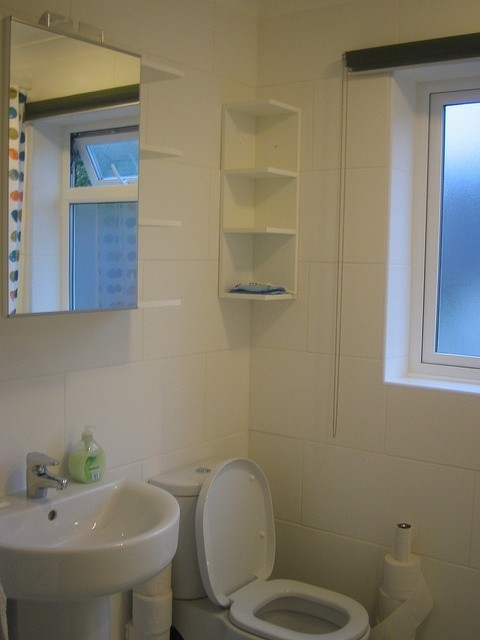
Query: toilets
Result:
<box><xmin>146</xmin><ymin>455</ymin><xmax>372</xmax><ymax>638</ymax></box>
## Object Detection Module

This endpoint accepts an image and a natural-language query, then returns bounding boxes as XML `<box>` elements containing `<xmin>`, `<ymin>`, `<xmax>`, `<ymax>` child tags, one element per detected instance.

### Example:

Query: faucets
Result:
<box><xmin>26</xmin><ymin>452</ymin><xmax>66</xmax><ymax>497</ymax></box>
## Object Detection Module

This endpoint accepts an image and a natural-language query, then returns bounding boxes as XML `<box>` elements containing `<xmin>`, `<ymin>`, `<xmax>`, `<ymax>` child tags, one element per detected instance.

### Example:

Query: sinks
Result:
<box><xmin>0</xmin><ymin>480</ymin><xmax>180</xmax><ymax>602</ymax></box>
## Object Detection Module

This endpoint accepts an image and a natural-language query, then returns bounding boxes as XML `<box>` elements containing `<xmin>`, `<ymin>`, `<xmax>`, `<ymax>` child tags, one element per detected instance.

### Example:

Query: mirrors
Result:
<box><xmin>5</xmin><ymin>19</ymin><xmax>141</xmax><ymax>315</ymax></box>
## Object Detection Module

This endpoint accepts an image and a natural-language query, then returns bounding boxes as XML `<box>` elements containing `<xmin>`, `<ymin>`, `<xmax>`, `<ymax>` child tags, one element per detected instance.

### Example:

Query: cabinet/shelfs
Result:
<box><xmin>216</xmin><ymin>97</ymin><xmax>303</xmax><ymax>304</ymax></box>
